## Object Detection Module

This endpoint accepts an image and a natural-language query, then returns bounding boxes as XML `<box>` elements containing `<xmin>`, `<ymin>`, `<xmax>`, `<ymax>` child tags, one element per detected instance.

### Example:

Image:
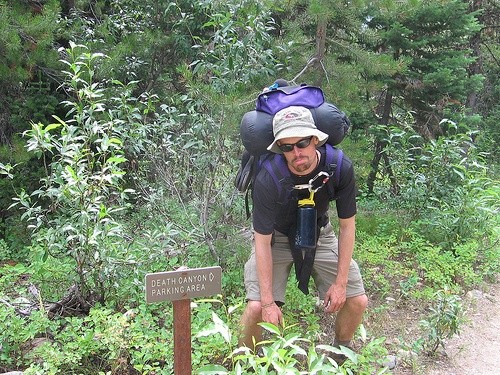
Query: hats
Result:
<box><xmin>267</xmin><ymin>106</ymin><xmax>329</xmax><ymax>154</ymax></box>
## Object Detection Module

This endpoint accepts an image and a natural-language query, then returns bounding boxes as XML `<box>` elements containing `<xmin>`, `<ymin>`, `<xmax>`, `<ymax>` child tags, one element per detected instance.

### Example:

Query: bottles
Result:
<box><xmin>294</xmin><ymin>190</ymin><xmax>317</xmax><ymax>250</ymax></box>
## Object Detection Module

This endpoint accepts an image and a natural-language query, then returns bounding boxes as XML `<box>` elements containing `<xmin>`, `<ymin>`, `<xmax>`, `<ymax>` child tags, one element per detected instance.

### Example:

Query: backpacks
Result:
<box><xmin>234</xmin><ymin>78</ymin><xmax>351</xmax><ymax>223</ymax></box>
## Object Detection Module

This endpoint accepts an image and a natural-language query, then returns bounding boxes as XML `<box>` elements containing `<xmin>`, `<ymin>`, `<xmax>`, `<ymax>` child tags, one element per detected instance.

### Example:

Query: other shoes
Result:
<box><xmin>328</xmin><ymin>346</ymin><xmax>355</xmax><ymax>366</ymax></box>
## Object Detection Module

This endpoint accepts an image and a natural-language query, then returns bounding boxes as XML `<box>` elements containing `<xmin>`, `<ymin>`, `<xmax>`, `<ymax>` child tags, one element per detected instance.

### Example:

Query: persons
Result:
<box><xmin>237</xmin><ymin>106</ymin><xmax>368</xmax><ymax>373</ymax></box>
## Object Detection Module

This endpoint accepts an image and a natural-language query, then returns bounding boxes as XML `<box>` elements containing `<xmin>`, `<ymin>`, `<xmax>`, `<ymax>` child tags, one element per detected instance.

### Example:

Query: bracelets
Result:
<box><xmin>261</xmin><ymin>301</ymin><xmax>275</xmax><ymax>308</ymax></box>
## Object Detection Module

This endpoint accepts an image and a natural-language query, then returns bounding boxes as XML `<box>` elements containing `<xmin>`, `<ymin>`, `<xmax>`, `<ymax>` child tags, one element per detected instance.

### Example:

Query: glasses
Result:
<box><xmin>275</xmin><ymin>136</ymin><xmax>314</xmax><ymax>152</ymax></box>
